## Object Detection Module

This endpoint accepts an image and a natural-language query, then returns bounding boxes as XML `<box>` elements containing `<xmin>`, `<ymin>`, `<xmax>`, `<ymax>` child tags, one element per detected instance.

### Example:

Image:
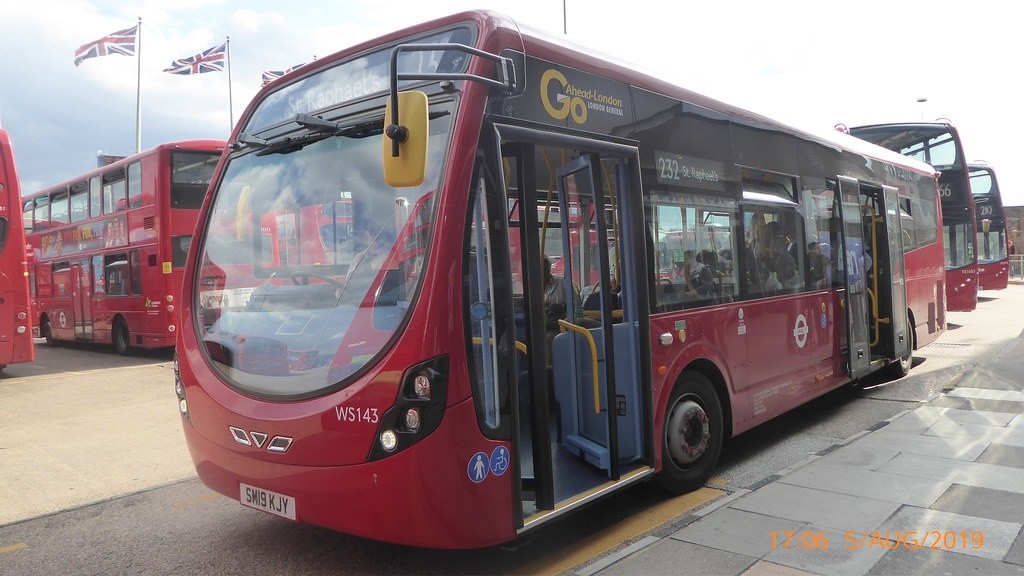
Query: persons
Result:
<box><xmin>538</xmin><ymin>212</ymin><xmax>836</xmax><ymax>331</ymax></box>
<box><xmin>334</xmin><ymin>218</ymin><xmax>393</xmax><ymax>300</ymax></box>
<box><xmin>1009</xmin><ymin>241</ymin><xmax>1015</xmax><ymax>276</ymax></box>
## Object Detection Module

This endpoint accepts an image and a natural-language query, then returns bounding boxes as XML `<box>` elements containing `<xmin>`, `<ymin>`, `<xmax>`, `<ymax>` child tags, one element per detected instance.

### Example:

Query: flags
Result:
<box><xmin>74</xmin><ymin>24</ymin><xmax>139</xmax><ymax>67</ymax></box>
<box><xmin>261</xmin><ymin>63</ymin><xmax>309</xmax><ymax>87</ymax></box>
<box><xmin>162</xmin><ymin>41</ymin><xmax>228</xmax><ymax>75</ymax></box>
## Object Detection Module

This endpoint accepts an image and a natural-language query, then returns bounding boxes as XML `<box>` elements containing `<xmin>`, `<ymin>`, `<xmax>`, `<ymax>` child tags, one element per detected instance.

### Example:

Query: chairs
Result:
<box><xmin>574</xmin><ymin>250</ymin><xmax>796</xmax><ymax>322</ymax></box>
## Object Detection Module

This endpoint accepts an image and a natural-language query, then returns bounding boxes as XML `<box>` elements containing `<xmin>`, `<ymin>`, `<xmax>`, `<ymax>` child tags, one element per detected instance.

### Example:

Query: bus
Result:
<box><xmin>18</xmin><ymin>140</ymin><xmax>279</xmax><ymax>356</ymax></box>
<box><xmin>264</xmin><ymin>189</ymin><xmax>355</xmax><ymax>290</ymax></box>
<box><xmin>0</xmin><ymin>127</ymin><xmax>37</xmax><ymax>373</ymax></box>
<box><xmin>826</xmin><ymin>116</ymin><xmax>992</xmax><ymax>315</ymax></box>
<box><xmin>932</xmin><ymin>161</ymin><xmax>1013</xmax><ymax>293</ymax></box>
<box><xmin>170</xmin><ymin>7</ymin><xmax>950</xmax><ymax>552</ymax></box>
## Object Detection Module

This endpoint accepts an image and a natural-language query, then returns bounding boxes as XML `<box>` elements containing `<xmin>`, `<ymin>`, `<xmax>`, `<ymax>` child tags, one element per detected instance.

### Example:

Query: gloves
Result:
<box><xmin>544</xmin><ymin>303</ymin><xmax>563</xmax><ymax>315</ymax></box>
